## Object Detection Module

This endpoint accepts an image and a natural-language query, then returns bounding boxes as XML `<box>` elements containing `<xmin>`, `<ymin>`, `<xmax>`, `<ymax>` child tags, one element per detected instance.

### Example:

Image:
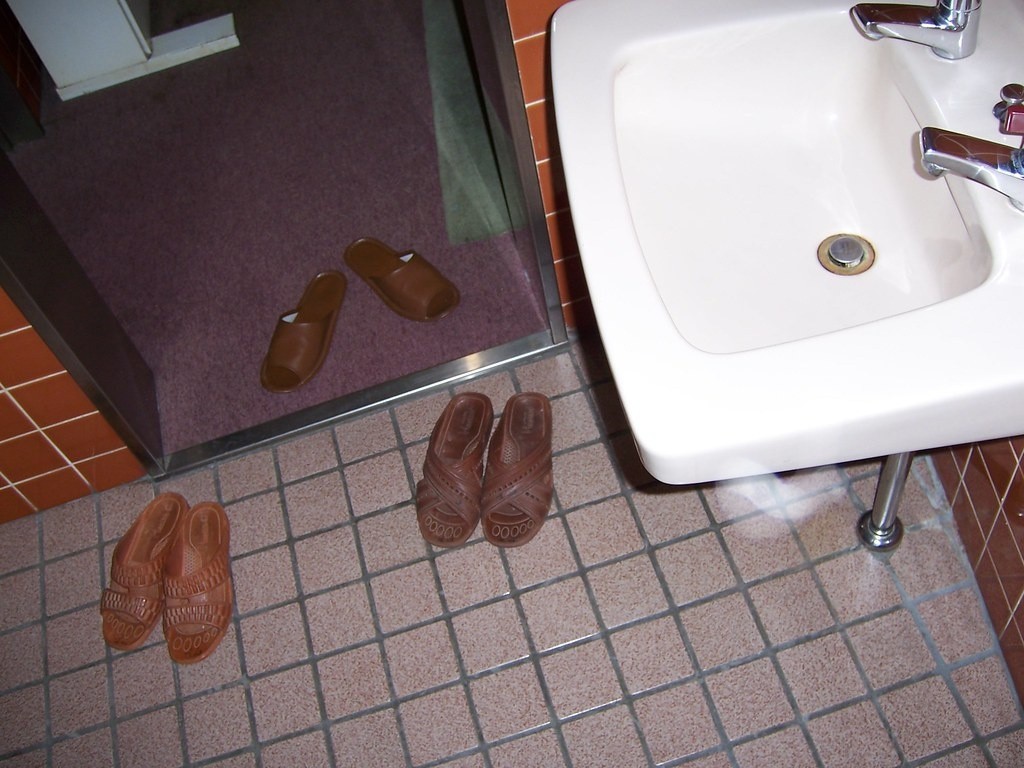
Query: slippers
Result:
<box><xmin>161</xmin><ymin>500</ymin><xmax>231</xmax><ymax>664</ymax></box>
<box><xmin>415</xmin><ymin>390</ymin><xmax>492</xmax><ymax>547</ymax></box>
<box><xmin>483</xmin><ymin>392</ymin><xmax>554</xmax><ymax>547</ymax></box>
<box><xmin>259</xmin><ymin>267</ymin><xmax>345</xmax><ymax>392</ymax></box>
<box><xmin>345</xmin><ymin>237</ymin><xmax>460</xmax><ymax>323</ymax></box>
<box><xmin>98</xmin><ymin>490</ymin><xmax>189</xmax><ymax>650</ymax></box>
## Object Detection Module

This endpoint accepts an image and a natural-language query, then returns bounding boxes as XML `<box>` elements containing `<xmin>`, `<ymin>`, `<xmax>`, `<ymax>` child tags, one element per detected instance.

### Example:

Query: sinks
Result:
<box><xmin>549</xmin><ymin>1</ymin><xmax>1023</xmax><ymax>484</ymax></box>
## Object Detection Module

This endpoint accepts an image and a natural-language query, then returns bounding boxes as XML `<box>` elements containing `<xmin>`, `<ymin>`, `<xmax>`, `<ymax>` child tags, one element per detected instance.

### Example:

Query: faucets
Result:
<box><xmin>848</xmin><ymin>1</ymin><xmax>982</xmax><ymax>62</ymax></box>
<box><xmin>919</xmin><ymin>107</ymin><xmax>1022</xmax><ymax>217</ymax></box>
